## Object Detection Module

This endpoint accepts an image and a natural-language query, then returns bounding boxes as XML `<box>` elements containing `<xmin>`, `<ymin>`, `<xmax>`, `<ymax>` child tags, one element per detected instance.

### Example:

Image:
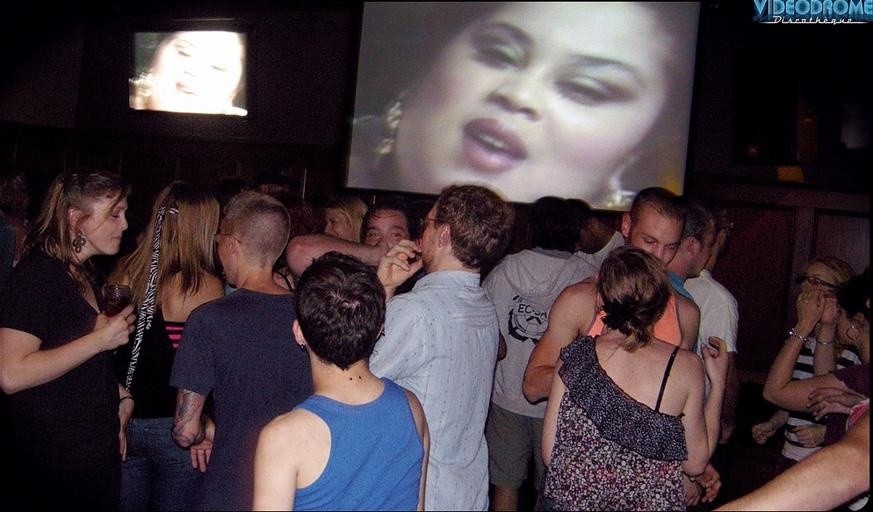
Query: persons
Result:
<box><xmin>347</xmin><ymin>0</ymin><xmax>696</xmax><ymax>215</ymax></box>
<box><xmin>0</xmin><ymin>166</ymin><xmax>871</xmax><ymax>512</ymax></box>
<box><xmin>128</xmin><ymin>29</ymin><xmax>249</xmax><ymax>121</ymax></box>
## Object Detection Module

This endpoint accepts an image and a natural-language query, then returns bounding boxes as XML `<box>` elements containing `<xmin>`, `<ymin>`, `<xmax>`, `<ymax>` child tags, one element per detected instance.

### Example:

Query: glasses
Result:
<box><xmin>419</xmin><ymin>218</ymin><xmax>455</xmax><ymax>237</ymax></box>
<box><xmin>794</xmin><ymin>272</ymin><xmax>836</xmax><ymax>289</ymax></box>
